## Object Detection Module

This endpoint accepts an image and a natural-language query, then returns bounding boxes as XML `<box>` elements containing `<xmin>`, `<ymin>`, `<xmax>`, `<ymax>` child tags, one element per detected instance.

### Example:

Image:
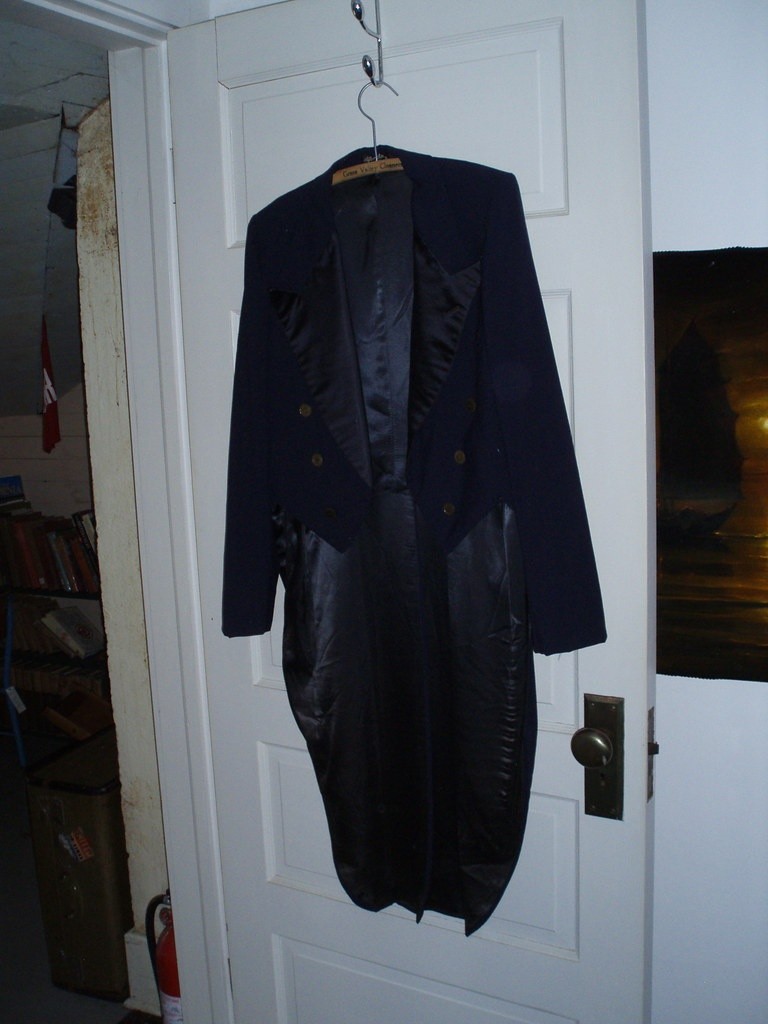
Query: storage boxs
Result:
<box><xmin>25</xmin><ymin>725</ymin><xmax>135</xmax><ymax>1003</ymax></box>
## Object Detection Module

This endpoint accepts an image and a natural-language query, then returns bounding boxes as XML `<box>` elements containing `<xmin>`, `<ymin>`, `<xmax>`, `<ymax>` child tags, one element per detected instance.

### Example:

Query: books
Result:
<box><xmin>0</xmin><ymin>474</ymin><xmax>112</xmax><ymax>737</ymax></box>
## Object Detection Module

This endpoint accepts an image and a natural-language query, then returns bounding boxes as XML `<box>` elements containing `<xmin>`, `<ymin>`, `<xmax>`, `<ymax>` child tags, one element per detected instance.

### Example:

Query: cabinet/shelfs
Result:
<box><xmin>0</xmin><ymin>584</ymin><xmax>116</xmax><ymax>740</ymax></box>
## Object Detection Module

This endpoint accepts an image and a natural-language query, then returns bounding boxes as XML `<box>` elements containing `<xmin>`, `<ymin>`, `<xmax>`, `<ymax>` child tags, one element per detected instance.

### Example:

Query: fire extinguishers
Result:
<box><xmin>145</xmin><ymin>890</ymin><xmax>182</xmax><ymax>1023</ymax></box>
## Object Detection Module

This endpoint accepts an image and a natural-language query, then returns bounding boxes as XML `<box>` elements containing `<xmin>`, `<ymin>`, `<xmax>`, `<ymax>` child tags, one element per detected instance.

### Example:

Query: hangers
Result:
<box><xmin>333</xmin><ymin>80</ymin><xmax>404</xmax><ymax>185</ymax></box>
